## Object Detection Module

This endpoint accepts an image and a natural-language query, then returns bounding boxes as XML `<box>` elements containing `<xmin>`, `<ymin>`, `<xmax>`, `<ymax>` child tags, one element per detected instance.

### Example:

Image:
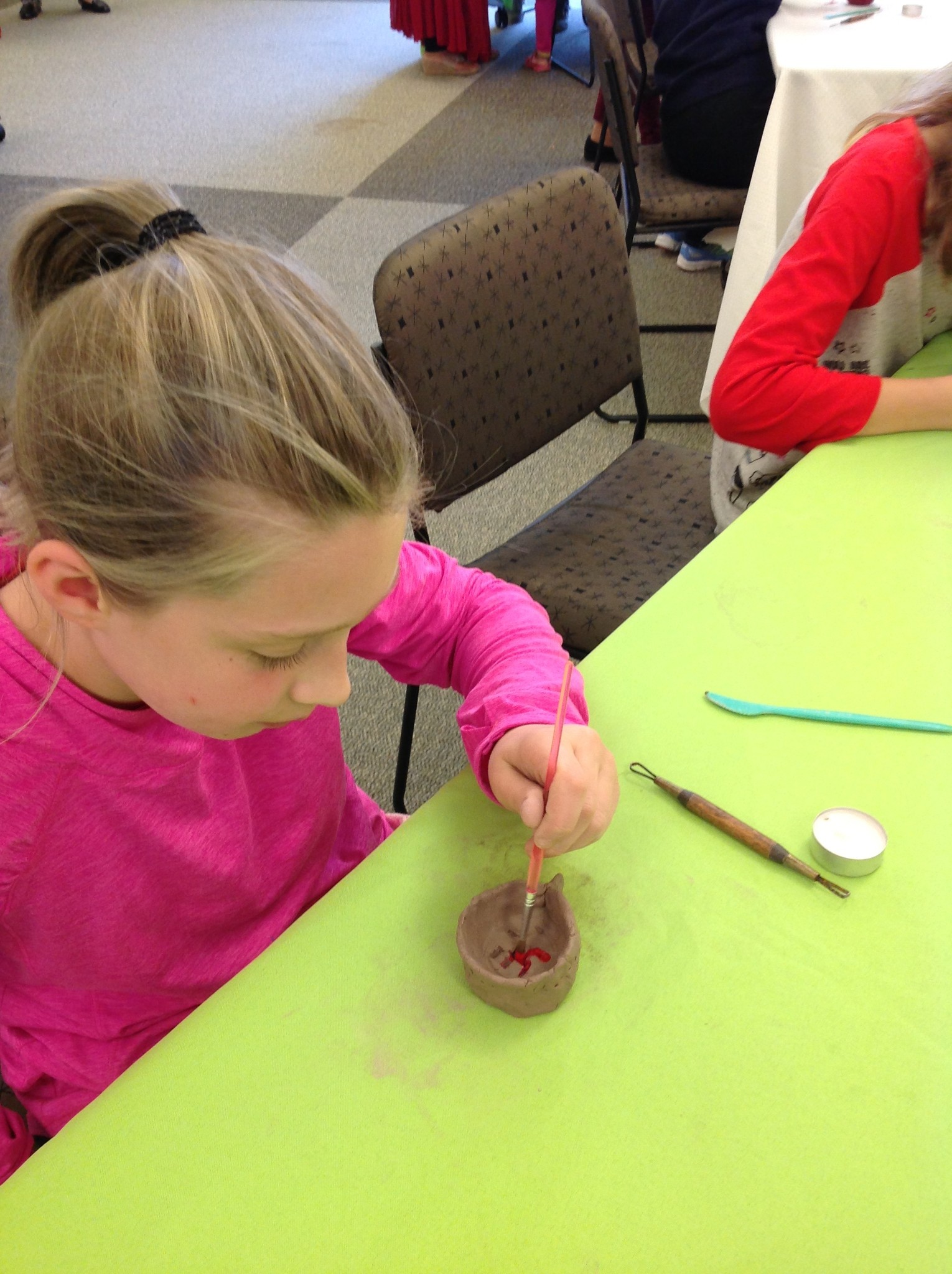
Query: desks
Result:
<box><xmin>0</xmin><ymin>329</ymin><xmax>952</xmax><ymax>1274</ymax></box>
<box><xmin>698</xmin><ymin>0</ymin><xmax>952</xmax><ymax>409</ymax></box>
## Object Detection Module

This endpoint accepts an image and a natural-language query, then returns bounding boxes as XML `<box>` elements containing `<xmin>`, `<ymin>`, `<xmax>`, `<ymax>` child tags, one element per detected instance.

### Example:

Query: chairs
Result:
<box><xmin>370</xmin><ymin>160</ymin><xmax>718</xmax><ymax>815</ymax></box>
<box><xmin>581</xmin><ymin>0</ymin><xmax>750</xmax><ymax>425</ymax></box>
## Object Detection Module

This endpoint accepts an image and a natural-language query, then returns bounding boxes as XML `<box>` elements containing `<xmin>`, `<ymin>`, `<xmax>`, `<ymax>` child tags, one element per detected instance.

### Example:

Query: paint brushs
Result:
<box><xmin>516</xmin><ymin>661</ymin><xmax>573</xmax><ymax>953</ymax></box>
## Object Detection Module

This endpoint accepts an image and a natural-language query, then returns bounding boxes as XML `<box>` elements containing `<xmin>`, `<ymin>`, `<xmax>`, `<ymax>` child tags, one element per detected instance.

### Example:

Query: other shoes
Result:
<box><xmin>551</xmin><ymin>8</ymin><xmax>570</xmax><ymax>35</ymax></box>
<box><xmin>19</xmin><ymin>0</ymin><xmax>42</xmax><ymax>20</ymax></box>
<box><xmin>584</xmin><ymin>135</ymin><xmax>618</xmax><ymax>164</ymax></box>
<box><xmin>78</xmin><ymin>0</ymin><xmax>111</xmax><ymax>13</ymax></box>
<box><xmin>526</xmin><ymin>51</ymin><xmax>553</xmax><ymax>73</ymax></box>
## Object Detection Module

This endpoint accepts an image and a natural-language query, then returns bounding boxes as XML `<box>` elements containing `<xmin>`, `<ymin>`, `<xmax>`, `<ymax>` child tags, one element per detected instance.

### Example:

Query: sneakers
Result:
<box><xmin>654</xmin><ymin>230</ymin><xmax>693</xmax><ymax>252</ymax></box>
<box><xmin>676</xmin><ymin>241</ymin><xmax>733</xmax><ymax>272</ymax></box>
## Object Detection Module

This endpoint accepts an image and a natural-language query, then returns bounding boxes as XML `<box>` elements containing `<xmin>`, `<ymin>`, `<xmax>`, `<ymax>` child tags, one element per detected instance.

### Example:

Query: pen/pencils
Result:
<box><xmin>826</xmin><ymin>8</ymin><xmax>880</xmax><ymax>19</ymax></box>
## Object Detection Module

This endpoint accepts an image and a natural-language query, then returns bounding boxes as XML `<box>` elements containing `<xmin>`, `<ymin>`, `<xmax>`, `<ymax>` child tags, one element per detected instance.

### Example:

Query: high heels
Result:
<box><xmin>489</xmin><ymin>46</ymin><xmax>500</xmax><ymax>61</ymax></box>
<box><xmin>421</xmin><ymin>49</ymin><xmax>481</xmax><ymax>76</ymax></box>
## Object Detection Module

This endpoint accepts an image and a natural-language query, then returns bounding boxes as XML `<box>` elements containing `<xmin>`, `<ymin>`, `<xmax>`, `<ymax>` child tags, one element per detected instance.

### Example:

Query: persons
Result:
<box><xmin>710</xmin><ymin>76</ymin><xmax>952</xmax><ymax>535</ymax></box>
<box><xmin>1</xmin><ymin>172</ymin><xmax>619</xmax><ymax>1188</ymax></box>
<box><xmin>20</xmin><ymin>0</ymin><xmax>111</xmax><ymax>19</ymax></box>
<box><xmin>390</xmin><ymin>0</ymin><xmax>570</xmax><ymax>75</ymax></box>
<box><xmin>583</xmin><ymin>0</ymin><xmax>776</xmax><ymax>270</ymax></box>
